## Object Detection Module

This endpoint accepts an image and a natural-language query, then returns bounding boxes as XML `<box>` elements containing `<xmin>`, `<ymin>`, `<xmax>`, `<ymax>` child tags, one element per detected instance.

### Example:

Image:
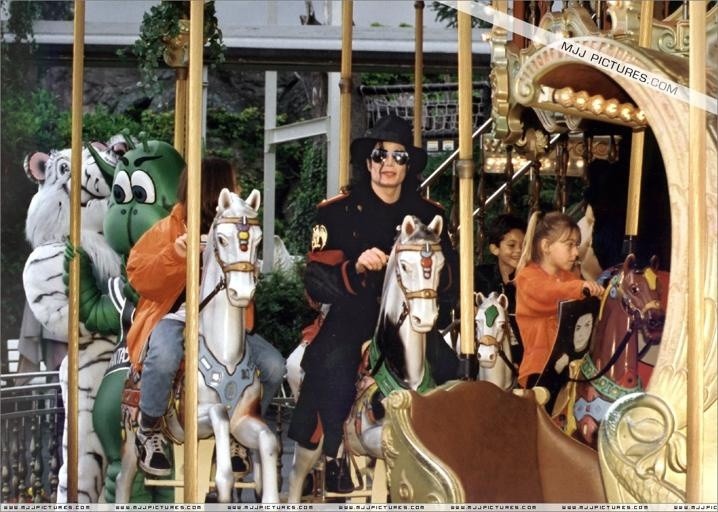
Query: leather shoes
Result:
<box><xmin>322</xmin><ymin>456</ymin><xmax>356</xmax><ymax>494</ymax></box>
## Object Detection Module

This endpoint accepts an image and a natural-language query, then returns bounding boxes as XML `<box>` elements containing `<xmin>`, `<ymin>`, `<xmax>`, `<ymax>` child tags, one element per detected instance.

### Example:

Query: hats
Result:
<box><xmin>350</xmin><ymin>115</ymin><xmax>428</xmax><ymax>174</ymax></box>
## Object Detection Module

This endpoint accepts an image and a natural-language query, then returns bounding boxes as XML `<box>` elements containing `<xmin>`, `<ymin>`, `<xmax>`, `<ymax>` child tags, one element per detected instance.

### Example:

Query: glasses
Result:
<box><xmin>368</xmin><ymin>148</ymin><xmax>409</xmax><ymax>166</ymax></box>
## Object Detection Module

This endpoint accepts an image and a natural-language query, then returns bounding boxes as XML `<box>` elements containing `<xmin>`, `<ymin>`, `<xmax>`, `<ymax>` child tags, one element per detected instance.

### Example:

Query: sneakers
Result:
<box><xmin>229</xmin><ymin>434</ymin><xmax>253</xmax><ymax>475</ymax></box>
<box><xmin>133</xmin><ymin>426</ymin><xmax>172</xmax><ymax>477</ymax></box>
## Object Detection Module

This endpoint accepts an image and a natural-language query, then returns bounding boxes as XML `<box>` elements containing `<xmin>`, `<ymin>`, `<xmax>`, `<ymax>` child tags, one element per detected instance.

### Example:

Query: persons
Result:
<box><xmin>474</xmin><ymin>213</ymin><xmax>527</xmax><ymax>372</ymax></box>
<box><xmin>63</xmin><ymin>127</ymin><xmax>188</xmax><ymax>503</ymax></box>
<box><xmin>553</xmin><ymin>303</ymin><xmax>595</xmax><ymax>382</ymax></box>
<box><xmin>516</xmin><ymin>207</ymin><xmax>604</xmax><ymax>405</ymax></box>
<box><xmin>303</xmin><ymin>115</ymin><xmax>464</xmax><ymax>491</ymax></box>
<box><xmin>126</xmin><ymin>156</ymin><xmax>283</xmax><ymax>482</ymax></box>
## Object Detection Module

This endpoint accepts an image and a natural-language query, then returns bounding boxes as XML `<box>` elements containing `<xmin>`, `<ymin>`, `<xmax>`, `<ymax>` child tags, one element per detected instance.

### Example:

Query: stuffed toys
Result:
<box><xmin>21</xmin><ymin>127</ymin><xmax>140</xmax><ymax>503</ymax></box>
